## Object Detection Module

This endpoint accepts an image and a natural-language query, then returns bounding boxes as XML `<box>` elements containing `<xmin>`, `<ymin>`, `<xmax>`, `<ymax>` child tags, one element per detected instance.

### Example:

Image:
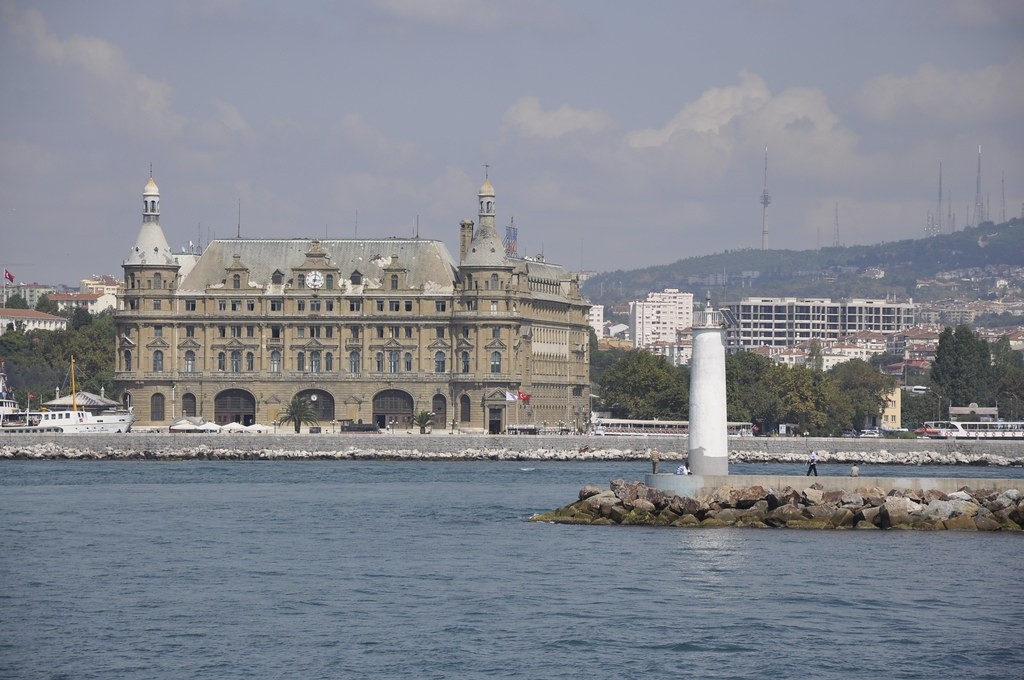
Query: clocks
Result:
<box><xmin>306</xmin><ymin>270</ymin><xmax>324</xmax><ymax>289</ymax></box>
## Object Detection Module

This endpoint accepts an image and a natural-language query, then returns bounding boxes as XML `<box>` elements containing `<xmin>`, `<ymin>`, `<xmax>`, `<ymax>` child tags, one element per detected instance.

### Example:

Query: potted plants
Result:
<box><xmin>274</xmin><ymin>396</ymin><xmax>319</xmax><ymax>433</ymax></box>
<box><xmin>408</xmin><ymin>409</ymin><xmax>436</xmax><ymax>435</ymax></box>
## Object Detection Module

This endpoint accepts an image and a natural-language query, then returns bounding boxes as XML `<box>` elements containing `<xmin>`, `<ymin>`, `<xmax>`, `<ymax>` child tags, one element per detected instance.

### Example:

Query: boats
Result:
<box><xmin>0</xmin><ymin>355</ymin><xmax>139</xmax><ymax>434</ymax></box>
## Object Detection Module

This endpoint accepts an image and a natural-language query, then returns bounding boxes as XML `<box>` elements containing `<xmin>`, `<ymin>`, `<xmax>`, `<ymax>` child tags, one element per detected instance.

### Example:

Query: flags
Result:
<box><xmin>5</xmin><ymin>269</ymin><xmax>15</xmax><ymax>283</ymax></box>
<box><xmin>517</xmin><ymin>390</ymin><xmax>531</xmax><ymax>401</ymax></box>
<box><xmin>505</xmin><ymin>390</ymin><xmax>518</xmax><ymax>401</ymax></box>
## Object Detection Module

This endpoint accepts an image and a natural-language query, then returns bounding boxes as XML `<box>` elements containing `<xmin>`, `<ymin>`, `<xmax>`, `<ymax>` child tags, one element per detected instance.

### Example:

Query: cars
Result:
<box><xmin>859</xmin><ymin>431</ymin><xmax>880</xmax><ymax>439</ymax></box>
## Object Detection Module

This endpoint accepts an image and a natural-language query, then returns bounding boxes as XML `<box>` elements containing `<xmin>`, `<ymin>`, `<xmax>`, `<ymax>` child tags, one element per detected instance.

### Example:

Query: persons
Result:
<box><xmin>850</xmin><ymin>464</ymin><xmax>859</xmax><ymax>477</ymax></box>
<box><xmin>806</xmin><ymin>448</ymin><xmax>817</xmax><ymax>476</ymax></box>
<box><xmin>649</xmin><ymin>447</ymin><xmax>659</xmax><ymax>474</ymax></box>
<box><xmin>751</xmin><ymin>424</ymin><xmax>760</xmax><ymax>437</ymax></box>
<box><xmin>674</xmin><ymin>459</ymin><xmax>690</xmax><ymax>475</ymax></box>
